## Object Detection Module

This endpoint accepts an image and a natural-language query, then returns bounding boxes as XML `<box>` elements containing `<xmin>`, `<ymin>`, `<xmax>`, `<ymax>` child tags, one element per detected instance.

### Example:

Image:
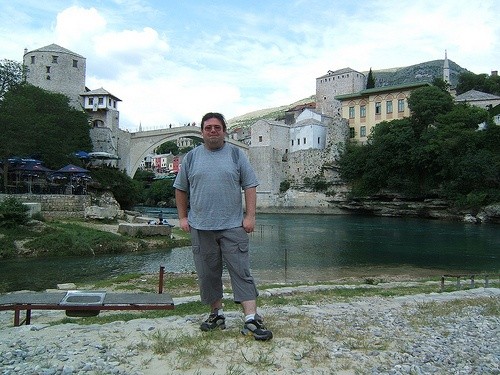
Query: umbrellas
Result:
<box><xmin>75</xmin><ymin>151</ymin><xmax>119</xmax><ymax>170</ymax></box>
<box><xmin>13</xmin><ymin>159</ymin><xmax>91</xmax><ymax>195</ymax></box>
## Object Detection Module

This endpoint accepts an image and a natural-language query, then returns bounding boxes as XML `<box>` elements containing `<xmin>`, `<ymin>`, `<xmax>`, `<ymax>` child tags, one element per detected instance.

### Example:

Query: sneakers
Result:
<box><xmin>199</xmin><ymin>308</ymin><xmax>226</xmax><ymax>332</ymax></box>
<box><xmin>240</xmin><ymin>314</ymin><xmax>273</xmax><ymax>340</ymax></box>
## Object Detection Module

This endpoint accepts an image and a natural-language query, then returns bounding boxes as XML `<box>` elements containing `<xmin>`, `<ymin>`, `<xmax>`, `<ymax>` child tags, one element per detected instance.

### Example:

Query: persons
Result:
<box><xmin>174</xmin><ymin>112</ymin><xmax>272</xmax><ymax>340</ymax></box>
<box><xmin>159</xmin><ymin>211</ymin><xmax>163</xmax><ymax>223</ymax></box>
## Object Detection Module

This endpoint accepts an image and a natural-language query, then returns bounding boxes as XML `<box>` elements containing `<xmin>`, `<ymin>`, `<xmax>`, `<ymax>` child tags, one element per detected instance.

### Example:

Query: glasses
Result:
<box><xmin>203</xmin><ymin>126</ymin><xmax>223</xmax><ymax>131</ymax></box>
